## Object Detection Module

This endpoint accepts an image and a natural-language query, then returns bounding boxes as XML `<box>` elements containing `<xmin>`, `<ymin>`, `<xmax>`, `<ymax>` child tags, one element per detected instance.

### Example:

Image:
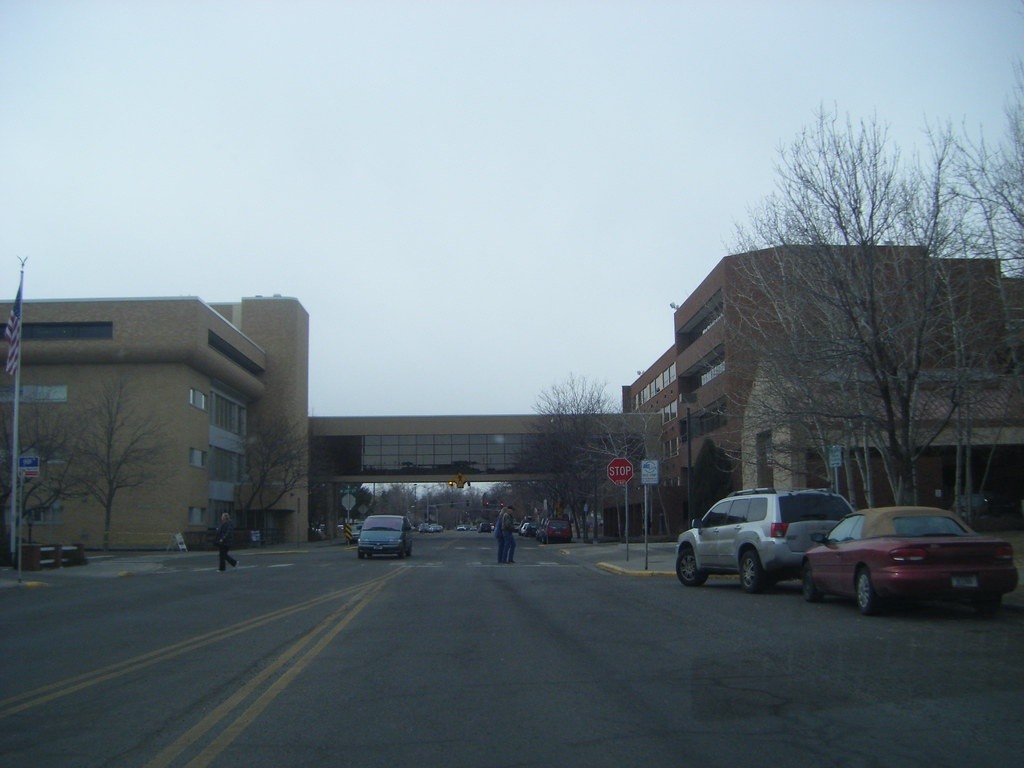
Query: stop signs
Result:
<box><xmin>607</xmin><ymin>457</ymin><xmax>633</xmax><ymax>486</ymax></box>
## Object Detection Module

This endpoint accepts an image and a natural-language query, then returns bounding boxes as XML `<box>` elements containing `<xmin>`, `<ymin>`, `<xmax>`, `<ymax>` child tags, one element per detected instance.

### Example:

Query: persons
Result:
<box><xmin>215</xmin><ymin>513</ymin><xmax>239</xmax><ymax>572</ymax></box>
<box><xmin>494</xmin><ymin>505</ymin><xmax>519</xmax><ymax>564</ymax></box>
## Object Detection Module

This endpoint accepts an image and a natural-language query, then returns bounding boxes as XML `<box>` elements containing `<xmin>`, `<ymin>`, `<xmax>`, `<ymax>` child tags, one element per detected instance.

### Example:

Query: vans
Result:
<box><xmin>358</xmin><ymin>515</ymin><xmax>413</xmax><ymax>560</ymax></box>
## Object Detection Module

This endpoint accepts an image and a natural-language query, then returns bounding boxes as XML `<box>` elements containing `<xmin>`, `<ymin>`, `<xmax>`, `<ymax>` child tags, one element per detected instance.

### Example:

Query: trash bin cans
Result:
<box><xmin>16</xmin><ymin>543</ymin><xmax>42</xmax><ymax>572</ymax></box>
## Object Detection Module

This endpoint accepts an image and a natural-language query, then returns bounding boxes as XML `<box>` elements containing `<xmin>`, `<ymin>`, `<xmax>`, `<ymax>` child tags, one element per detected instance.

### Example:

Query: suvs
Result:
<box><xmin>676</xmin><ymin>486</ymin><xmax>853</xmax><ymax>594</ymax></box>
<box><xmin>513</xmin><ymin>516</ymin><xmax>573</xmax><ymax>545</ymax></box>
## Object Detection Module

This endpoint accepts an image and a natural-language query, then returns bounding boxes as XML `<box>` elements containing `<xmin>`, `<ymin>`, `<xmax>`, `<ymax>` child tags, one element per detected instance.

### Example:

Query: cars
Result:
<box><xmin>416</xmin><ymin>523</ymin><xmax>443</xmax><ymax>533</ymax></box>
<box><xmin>457</xmin><ymin>522</ymin><xmax>495</xmax><ymax>533</ymax></box>
<box><xmin>800</xmin><ymin>504</ymin><xmax>1020</xmax><ymax>618</ymax></box>
<box><xmin>312</xmin><ymin>523</ymin><xmax>360</xmax><ymax>544</ymax></box>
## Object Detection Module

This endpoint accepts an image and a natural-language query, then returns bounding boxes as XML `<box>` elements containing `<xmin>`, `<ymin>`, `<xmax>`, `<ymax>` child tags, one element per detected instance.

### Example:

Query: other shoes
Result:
<box><xmin>233</xmin><ymin>561</ymin><xmax>239</xmax><ymax>569</ymax></box>
<box><xmin>509</xmin><ymin>560</ymin><xmax>515</xmax><ymax>563</ymax></box>
<box><xmin>216</xmin><ymin>569</ymin><xmax>226</xmax><ymax>572</ymax></box>
<box><xmin>502</xmin><ymin>561</ymin><xmax>510</xmax><ymax>564</ymax></box>
